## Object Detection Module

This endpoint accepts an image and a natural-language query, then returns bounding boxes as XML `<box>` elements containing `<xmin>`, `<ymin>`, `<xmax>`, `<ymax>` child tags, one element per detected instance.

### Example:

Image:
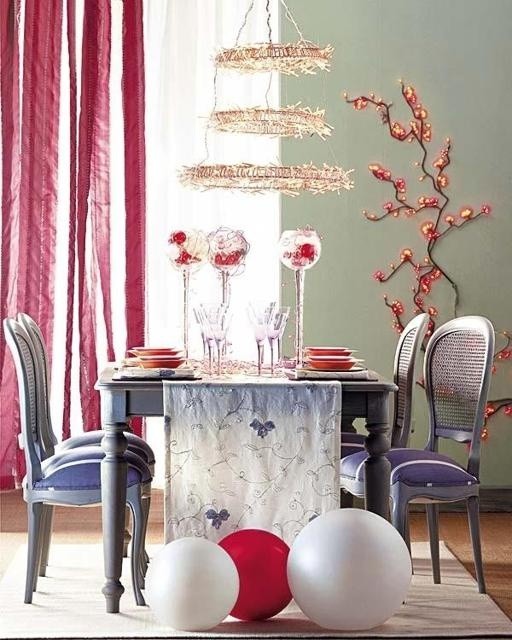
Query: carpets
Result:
<box><xmin>0</xmin><ymin>538</ymin><xmax>511</xmax><ymax>638</ymax></box>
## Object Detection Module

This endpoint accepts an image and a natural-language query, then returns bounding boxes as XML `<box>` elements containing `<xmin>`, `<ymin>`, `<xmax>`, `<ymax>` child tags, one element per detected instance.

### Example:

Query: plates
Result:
<box><xmin>126</xmin><ymin>347</ymin><xmax>186</xmax><ymax>368</ymax></box>
<box><xmin>304</xmin><ymin>346</ymin><xmax>358</xmax><ymax>370</ymax></box>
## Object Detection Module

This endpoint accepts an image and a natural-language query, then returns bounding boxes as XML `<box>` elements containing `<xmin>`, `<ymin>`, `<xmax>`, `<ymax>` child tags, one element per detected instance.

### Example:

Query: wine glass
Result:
<box><xmin>192</xmin><ymin>302</ymin><xmax>234</xmax><ymax>382</ymax></box>
<box><xmin>249</xmin><ymin>303</ymin><xmax>291</xmax><ymax>379</ymax></box>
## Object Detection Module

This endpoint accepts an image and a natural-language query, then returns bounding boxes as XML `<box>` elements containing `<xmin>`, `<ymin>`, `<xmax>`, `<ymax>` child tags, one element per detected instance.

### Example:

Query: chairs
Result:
<box><xmin>340</xmin><ymin>313</ymin><xmax>430</xmax><ymax>507</ymax></box>
<box><xmin>18</xmin><ymin>312</ymin><xmax>156</xmax><ymax>578</ymax></box>
<box><xmin>2</xmin><ymin>318</ymin><xmax>152</xmax><ymax>606</ymax></box>
<box><xmin>340</xmin><ymin>316</ymin><xmax>496</xmax><ymax>596</ymax></box>
